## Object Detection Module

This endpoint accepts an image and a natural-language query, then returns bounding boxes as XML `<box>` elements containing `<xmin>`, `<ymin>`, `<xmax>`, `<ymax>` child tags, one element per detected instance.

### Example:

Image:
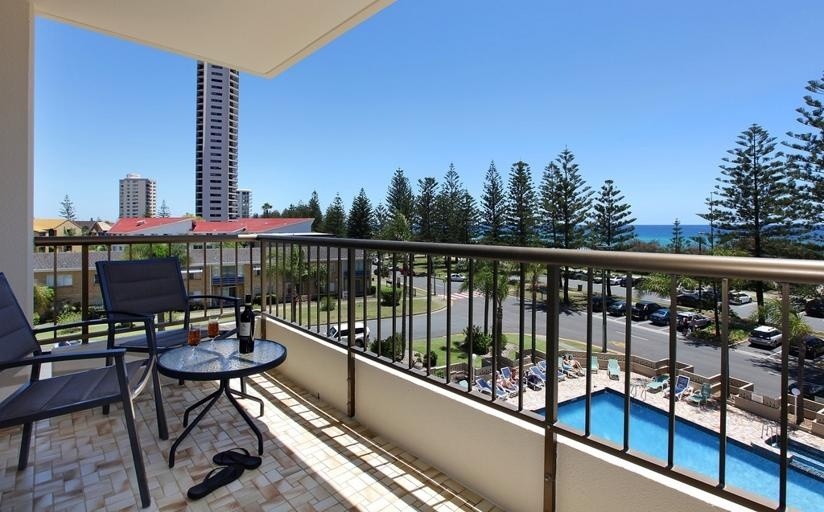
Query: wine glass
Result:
<box><xmin>187</xmin><ymin>322</ymin><xmax>201</xmax><ymax>354</ymax></box>
<box><xmin>207</xmin><ymin>318</ymin><xmax>220</xmax><ymax>349</ymax></box>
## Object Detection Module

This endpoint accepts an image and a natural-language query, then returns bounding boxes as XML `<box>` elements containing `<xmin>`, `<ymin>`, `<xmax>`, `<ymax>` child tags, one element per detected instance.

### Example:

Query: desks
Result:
<box><xmin>157</xmin><ymin>336</ymin><xmax>287</xmax><ymax>468</ymax></box>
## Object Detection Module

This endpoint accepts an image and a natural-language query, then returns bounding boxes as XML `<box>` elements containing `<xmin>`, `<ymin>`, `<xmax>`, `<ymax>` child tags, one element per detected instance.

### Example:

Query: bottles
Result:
<box><xmin>238</xmin><ymin>294</ymin><xmax>255</xmax><ymax>354</ymax></box>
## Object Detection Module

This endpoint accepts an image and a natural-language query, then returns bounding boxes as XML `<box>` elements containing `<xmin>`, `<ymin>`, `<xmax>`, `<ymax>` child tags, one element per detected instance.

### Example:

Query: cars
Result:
<box><xmin>779</xmin><ymin>296</ymin><xmax>824</xmax><ymax>317</ymax></box>
<box><xmin>323</xmin><ymin>321</ymin><xmax>370</xmax><ymax>348</ymax></box>
<box><xmin>729</xmin><ymin>291</ymin><xmax>753</xmax><ymax>305</ymax></box>
<box><xmin>748</xmin><ymin>321</ymin><xmax>824</xmax><ymax>359</ymax></box>
<box><xmin>372</xmin><ymin>257</ymin><xmax>416</xmax><ymax>278</ymax></box>
<box><xmin>450</xmin><ymin>273</ymin><xmax>466</xmax><ymax>282</ymax></box>
<box><xmin>789</xmin><ymin>374</ymin><xmax>824</xmax><ymax>399</ymax></box>
<box><xmin>560</xmin><ymin>267</ymin><xmax>719</xmax><ymax>330</ymax></box>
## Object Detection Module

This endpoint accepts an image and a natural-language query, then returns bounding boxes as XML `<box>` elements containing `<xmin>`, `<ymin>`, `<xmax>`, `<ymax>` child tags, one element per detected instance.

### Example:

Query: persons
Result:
<box><xmin>663</xmin><ymin>379</ymin><xmax>670</xmax><ymax>398</ymax></box>
<box><xmin>563</xmin><ymin>355</ymin><xmax>582</xmax><ymax>371</ymax></box>
<box><xmin>496</xmin><ymin>371</ymin><xmax>514</xmax><ymax>390</ymax></box>
<box><xmin>683</xmin><ymin>319</ymin><xmax>690</xmax><ymax>336</ymax></box>
<box><xmin>509</xmin><ymin>368</ymin><xmax>520</xmax><ymax>384</ymax></box>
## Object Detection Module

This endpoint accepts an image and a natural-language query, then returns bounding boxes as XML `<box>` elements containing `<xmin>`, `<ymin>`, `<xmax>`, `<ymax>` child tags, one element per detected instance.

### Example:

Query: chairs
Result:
<box><xmin>94</xmin><ymin>256</ymin><xmax>241</xmax><ymax>440</ymax></box>
<box><xmin>1</xmin><ymin>271</ymin><xmax>168</xmax><ymax>509</ymax></box>
<box><xmin>451</xmin><ymin>356</ymin><xmax>712</xmax><ymax>409</ymax></box>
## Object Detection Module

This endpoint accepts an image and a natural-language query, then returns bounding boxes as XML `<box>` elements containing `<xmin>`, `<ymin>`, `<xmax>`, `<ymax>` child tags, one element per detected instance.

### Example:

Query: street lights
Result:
<box><xmin>472</xmin><ymin>353</ymin><xmax>477</xmax><ymax>382</ymax></box>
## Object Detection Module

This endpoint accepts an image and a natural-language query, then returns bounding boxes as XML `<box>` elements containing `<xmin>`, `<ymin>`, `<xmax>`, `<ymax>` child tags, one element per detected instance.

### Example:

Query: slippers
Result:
<box><xmin>186</xmin><ymin>463</ymin><xmax>245</xmax><ymax>500</ymax></box>
<box><xmin>212</xmin><ymin>446</ymin><xmax>262</xmax><ymax>471</ymax></box>
